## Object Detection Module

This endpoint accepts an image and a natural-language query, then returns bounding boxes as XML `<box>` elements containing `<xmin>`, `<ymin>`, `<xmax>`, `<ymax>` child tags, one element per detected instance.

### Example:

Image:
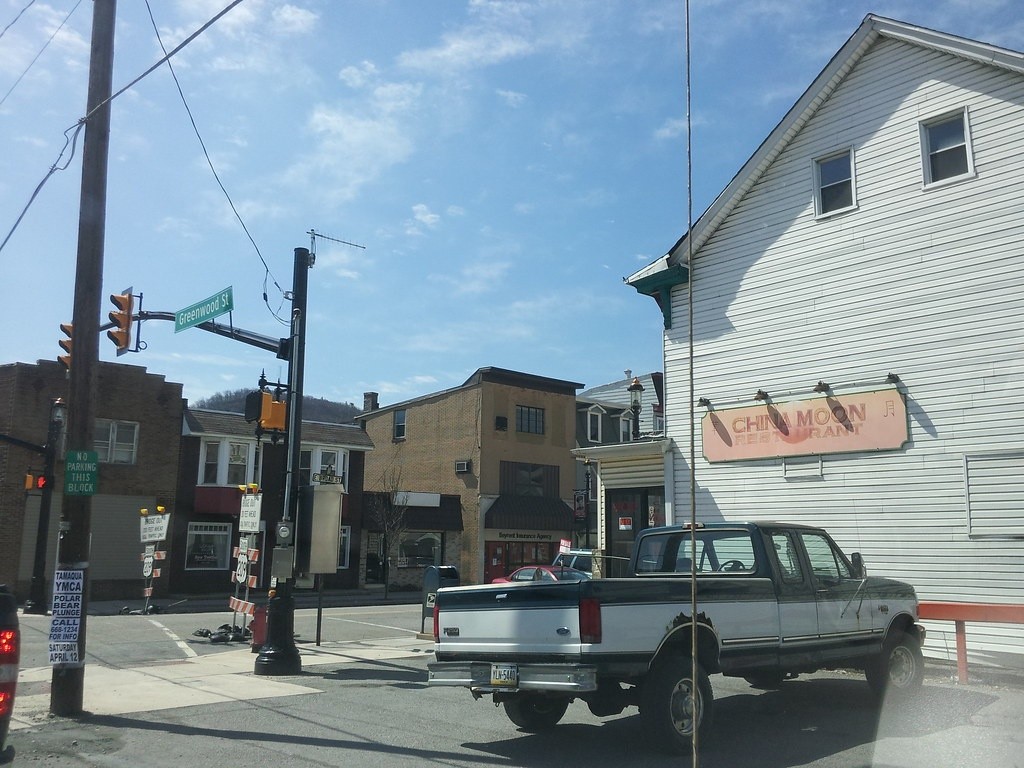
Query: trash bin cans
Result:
<box><xmin>337</xmin><ymin>569</ymin><xmax>353</xmax><ymax>589</ymax></box>
<box><xmin>422</xmin><ymin>565</ymin><xmax>460</xmax><ymax>620</ymax></box>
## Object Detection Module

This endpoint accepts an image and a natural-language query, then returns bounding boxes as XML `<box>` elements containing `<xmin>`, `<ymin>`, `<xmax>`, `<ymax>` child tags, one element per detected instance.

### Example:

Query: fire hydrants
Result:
<box><xmin>247</xmin><ymin>602</ymin><xmax>268</xmax><ymax>654</ymax></box>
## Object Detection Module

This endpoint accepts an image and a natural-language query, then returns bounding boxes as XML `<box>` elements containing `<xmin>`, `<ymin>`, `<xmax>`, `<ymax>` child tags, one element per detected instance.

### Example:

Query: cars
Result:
<box><xmin>491</xmin><ymin>563</ymin><xmax>589</xmax><ymax>584</ymax></box>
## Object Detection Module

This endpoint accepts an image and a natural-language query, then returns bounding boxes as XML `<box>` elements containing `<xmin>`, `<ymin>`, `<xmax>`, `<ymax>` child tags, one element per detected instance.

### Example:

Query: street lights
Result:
<box><xmin>22</xmin><ymin>396</ymin><xmax>66</xmax><ymax>614</ymax></box>
<box><xmin>583</xmin><ymin>455</ymin><xmax>595</xmax><ymax>549</ymax></box>
<box><xmin>626</xmin><ymin>376</ymin><xmax>649</xmax><ymax>538</ymax></box>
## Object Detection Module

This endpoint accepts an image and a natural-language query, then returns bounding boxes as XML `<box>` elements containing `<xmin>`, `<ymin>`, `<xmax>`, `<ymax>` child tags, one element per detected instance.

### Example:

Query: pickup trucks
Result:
<box><xmin>425</xmin><ymin>518</ymin><xmax>927</xmax><ymax>759</ymax></box>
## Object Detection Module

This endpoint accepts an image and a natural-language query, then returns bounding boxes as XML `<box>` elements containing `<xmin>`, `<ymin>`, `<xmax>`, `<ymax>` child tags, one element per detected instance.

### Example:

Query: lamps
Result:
<box><xmin>697</xmin><ymin>397</ymin><xmax>710</xmax><ymax>407</ymax></box>
<box><xmin>626</xmin><ymin>376</ymin><xmax>646</xmax><ymax>413</ymax></box>
<box><xmin>885</xmin><ymin>372</ymin><xmax>899</xmax><ymax>385</ymax></box>
<box><xmin>754</xmin><ymin>389</ymin><xmax>768</xmax><ymax>402</ymax></box>
<box><xmin>813</xmin><ymin>381</ymin><xmax>830</xmax><ymax>393</ymax></box>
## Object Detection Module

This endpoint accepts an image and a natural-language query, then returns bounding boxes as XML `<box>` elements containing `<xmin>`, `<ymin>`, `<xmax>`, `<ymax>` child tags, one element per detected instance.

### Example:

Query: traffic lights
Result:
<box><xmin>56</xmin><ymin>320</ymin><xmax>73</xmax><ymax>379</ymax></box>
<box><xmin>108</xmin><ymin>285</ymin><xmax>143</xmax><ymax>357</ymax></box>
<box><xmin>243</xmin><ymin>389</ymin><xmax>273</xmax><ymax>422</ymax></box>
<box><xmin>37</xmin><ymin>476</ymin><xmax>53</xmax><ymax>490</ymax></box>
<box><xmin>24</xmin><ymin>474</ymin><xmax>33</xmax><ymax>491</ymax></box>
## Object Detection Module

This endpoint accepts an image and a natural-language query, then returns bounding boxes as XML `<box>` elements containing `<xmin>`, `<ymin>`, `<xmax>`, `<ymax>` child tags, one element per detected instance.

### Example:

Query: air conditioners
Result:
<box><xmin>455</xmin><ymin>462</ymin><xmax>468</xmax><ymax>472</ymax></box>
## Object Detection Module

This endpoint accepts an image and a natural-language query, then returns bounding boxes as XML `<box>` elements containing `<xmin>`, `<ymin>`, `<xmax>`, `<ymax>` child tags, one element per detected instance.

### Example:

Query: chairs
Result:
<box><xmin>675</xmin><ymin>558</ymin><xmax>700</xmax><ymax>573</ymax></box>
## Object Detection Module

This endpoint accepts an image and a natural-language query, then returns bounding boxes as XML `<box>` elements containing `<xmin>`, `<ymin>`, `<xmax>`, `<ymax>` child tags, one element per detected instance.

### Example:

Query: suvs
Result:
<box><xmin>549</xmin><ymin>548</ymin><xmax>594</xmax><ymax>579</ymax></box>
